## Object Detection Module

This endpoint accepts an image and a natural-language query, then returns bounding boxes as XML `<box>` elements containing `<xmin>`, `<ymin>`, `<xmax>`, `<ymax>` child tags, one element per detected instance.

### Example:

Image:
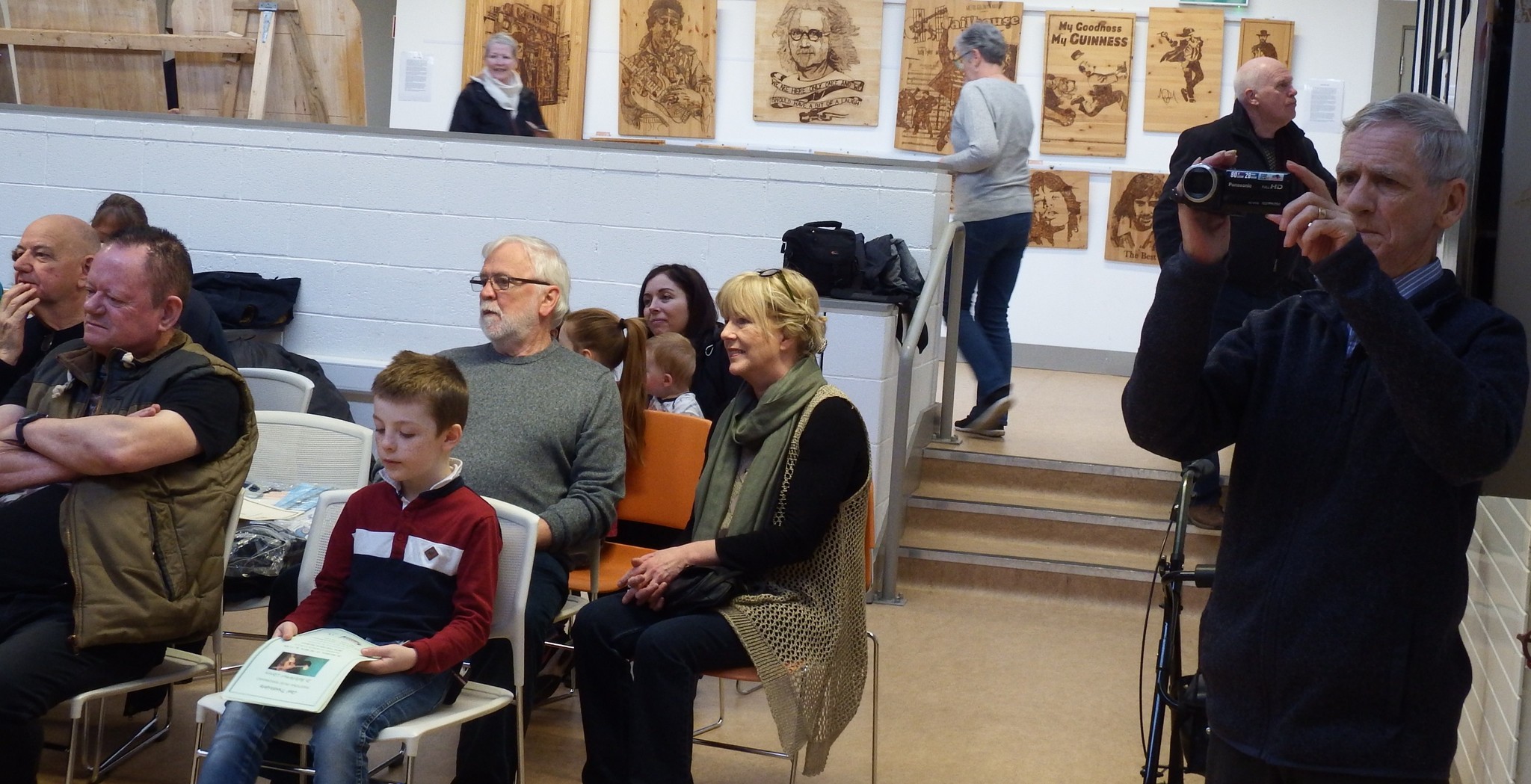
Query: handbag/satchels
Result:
<box><xmin>780</xmin><ymin>221</ymin><xmax>856</xmax><ymax>287</ymax></box>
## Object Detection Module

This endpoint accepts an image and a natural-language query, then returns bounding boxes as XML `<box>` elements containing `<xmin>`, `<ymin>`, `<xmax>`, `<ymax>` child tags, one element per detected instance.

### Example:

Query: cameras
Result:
<box><xmin>1175</xmin><ymin>161</ymin><xmax>1295</xmax><ymax>219</ymax></box>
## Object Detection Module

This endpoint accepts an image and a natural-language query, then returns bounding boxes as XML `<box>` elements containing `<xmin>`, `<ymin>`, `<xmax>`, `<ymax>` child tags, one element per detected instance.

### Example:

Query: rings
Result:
<box><xmin>1318</xmin><ymin>206</ymin><xmax>1326</xmax><ymax>220</ymax></box>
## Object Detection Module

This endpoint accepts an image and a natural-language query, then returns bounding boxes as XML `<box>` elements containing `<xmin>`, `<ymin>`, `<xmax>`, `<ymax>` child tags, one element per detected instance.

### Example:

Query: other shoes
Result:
<box><xmin>968</xmin><ymin>383</ymin><xmax>1018</xmax><ymax>435</ymax></box>
<box><xmin>1188</xmin><ymin>503</ymin><xmax>1226</xmax><ymax>531</ymax></box>
<box><xmin>954</xmin><ymin>405</ymin><xmax>1006</xmax><ymax>437</ymax></box>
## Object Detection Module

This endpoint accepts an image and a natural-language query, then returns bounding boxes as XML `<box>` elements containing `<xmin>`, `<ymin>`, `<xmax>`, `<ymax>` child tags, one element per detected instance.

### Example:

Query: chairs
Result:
<box><xmin>235</xmin><ymin>367</ymin><xmax>317</xmax><ymax>412</ymax></box>
<box><xmin>566</xmin><ymin>408</ymin><xmax>713</xmax><ymax>595</ymax></box>
<box><xmin>43</xmin><ymin>488</ymin><xmax>250</xmax><ymax>784</ymax></box>
<box><xmin>622</xmin><ymin>479</ymin><xmax>879</xmax><ymax>784</ymax></box>
<box><xmin>191</xmin><ymin>489</ymin><xmax>540</xmax><ymax>784</ymax></box>
<box><xmin>191</xmin><ymin>408</ymin><xmax>375</xmax><ymax>682</ymax></box>
<box><xmin>534</xmin><ymin>534</ymin><xmax>603</xmax><ymax>703</ymax></box>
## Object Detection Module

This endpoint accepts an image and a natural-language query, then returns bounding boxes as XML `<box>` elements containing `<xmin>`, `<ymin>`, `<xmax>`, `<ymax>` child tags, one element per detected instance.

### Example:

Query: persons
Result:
<box><xmin>449</xmin><ymin>31</ymin><xmax>556</xmax><ymax>139</ymax></box>
<box><xmin>0</xmin><ymin>193</ymin><xmax>873</xmax><ymax>784</ymax></box>
<box><xmin>1153</xmin><ymin>56</ymin><xmax>1337</xmax><ymax>528</ymax></box>
<box><xmin>942</xmin><ymin>23</ymin><xmax>1035</xmax><ymax>435</ymax></box>
<box><xmin>1121</xmin><ymin>92</ymin><xmax>1530</xmax><ymax>784</ymax></box>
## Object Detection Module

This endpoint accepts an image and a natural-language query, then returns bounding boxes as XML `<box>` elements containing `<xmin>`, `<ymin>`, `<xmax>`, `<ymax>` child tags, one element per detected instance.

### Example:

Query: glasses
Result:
<box><xmin>754</xmin><ymin>268</ymin><xmax>796</xmax><ymax>303</ymax></box>
<box><xmin>469</xmin><ymin>274</ymin><xmax>551</xmax><ymax>292</ymax></box>
<box><xmin>952</xmin><ymin>50</ymin><xmax>972</xmax><ymax>69</ymax></box>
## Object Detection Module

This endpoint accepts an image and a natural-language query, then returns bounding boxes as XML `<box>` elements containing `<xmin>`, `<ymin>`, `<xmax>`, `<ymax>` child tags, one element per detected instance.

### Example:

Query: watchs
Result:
<box><xmin>17</xmin><ymin>412</ymin><xmax>48</xmax><ymax>448</ymax></box>
